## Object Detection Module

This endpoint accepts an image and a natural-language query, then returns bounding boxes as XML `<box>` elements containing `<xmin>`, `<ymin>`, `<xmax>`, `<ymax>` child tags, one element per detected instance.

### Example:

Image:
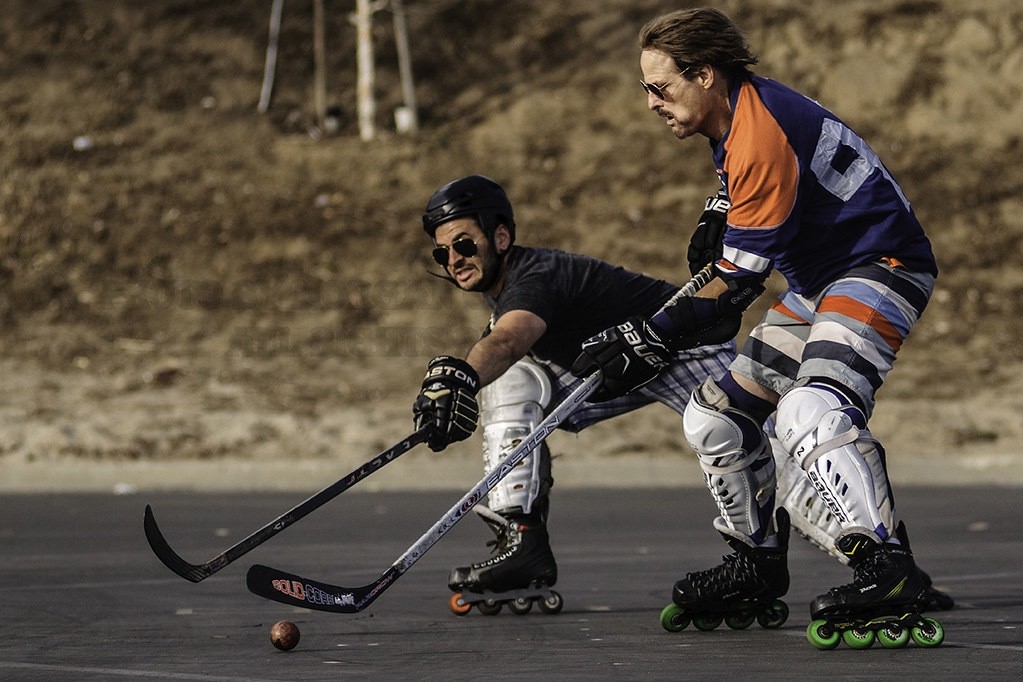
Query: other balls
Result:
<box><xmin>270</xmin><ymin>621</ymin><xmax>300</xmax><ymax>650</ymax></box>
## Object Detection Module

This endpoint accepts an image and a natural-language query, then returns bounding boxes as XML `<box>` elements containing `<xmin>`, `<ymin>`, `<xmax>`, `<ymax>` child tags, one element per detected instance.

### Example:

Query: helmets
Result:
<box><xmin>421</xmin><ymin>174</ymin><xmax>516</xmax><ymax>255</ymax></box>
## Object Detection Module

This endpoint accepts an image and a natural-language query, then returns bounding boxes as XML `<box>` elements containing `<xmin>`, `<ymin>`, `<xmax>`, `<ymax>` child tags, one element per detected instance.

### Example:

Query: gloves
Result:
<box><xmin>570</xmin><ymin>316</ymin><xmax>679</xmax><ymax>404</ymax></box>
<box><xmin>414</xmin><ymin>356</ymin><xmax>481</xmax><ymax>449</ymax></box>
<box><xmin>687</xmin><ymin>188</ymin><xmax>731</xmax><ymax>281</ymax></box>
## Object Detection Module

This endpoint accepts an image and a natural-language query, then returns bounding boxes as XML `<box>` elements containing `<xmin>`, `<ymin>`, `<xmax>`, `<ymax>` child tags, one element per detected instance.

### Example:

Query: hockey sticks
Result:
<box><xmin>143</xmin><ymin>423</ymin><xmax>432</xmax><ymax>582</ymax></box>
<box><xmin>246</xmin><ymin>369</ymin><xmax>606</xmax><ymax>615</ymax></box>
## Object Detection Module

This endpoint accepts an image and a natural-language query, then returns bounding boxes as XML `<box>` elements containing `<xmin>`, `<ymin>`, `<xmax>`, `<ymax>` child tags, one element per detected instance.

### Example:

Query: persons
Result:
<box><xmin>570</xmin><ymin>7</ymin><xmax>944</xmax><ymax>648</ymax></box>
<box><xmin>409</xmin><ymin>174</ymin><xmax>779</xmax><ymax>616</ymax></box>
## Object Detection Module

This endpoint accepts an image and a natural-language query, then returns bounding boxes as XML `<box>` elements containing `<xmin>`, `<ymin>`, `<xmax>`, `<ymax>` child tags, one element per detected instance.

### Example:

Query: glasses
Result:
<box><xmin>639</xmin><ymin>63</ymin><xmax>695</xmax><ymax>100</ymax></box>
<box><xmin>433</xmin><ymin>231</ymin><xmax>487</xmax><ymax>266</ymax></box>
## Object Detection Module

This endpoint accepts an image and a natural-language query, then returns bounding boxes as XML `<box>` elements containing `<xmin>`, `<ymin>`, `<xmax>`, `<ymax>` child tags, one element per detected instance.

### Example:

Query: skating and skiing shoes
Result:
<box><xmin>659</xmin><ymin>506</ymin><xmax>792</xmax><ymax>633</ymax></box>
<box><xmin>892</xmin><ymin>519</ymin><xmax>950</xmax><ymax>610</ymax></box>
<box><xmin>448</xmin><ymin>483</ymin><xmax>565</xmax><ymax>614</ymax></box>
<box><xmin>806</xmin><ymin>521</ymin><xmax>945</xmax><ymax>649</ymax></box>
